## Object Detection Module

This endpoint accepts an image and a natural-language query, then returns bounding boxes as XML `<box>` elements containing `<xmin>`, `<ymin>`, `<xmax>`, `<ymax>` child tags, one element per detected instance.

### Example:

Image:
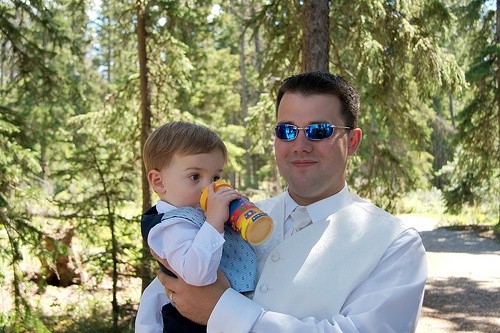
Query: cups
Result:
<box><xmin>199</xmin><ymin>179</ymin><xmax>274</xmax><ymax>246</ymax></box>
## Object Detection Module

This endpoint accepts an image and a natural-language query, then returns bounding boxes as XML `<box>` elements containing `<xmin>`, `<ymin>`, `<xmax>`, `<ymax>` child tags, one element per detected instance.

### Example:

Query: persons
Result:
<box><xmin>138</xmin><ymin>122</ymin><xmax>259</xmax><ymax>333</ymax></box>
<box><xmin>134</xmin><ymin>71</ymin><xmax>429</xmax><ymax>333</ymax></box>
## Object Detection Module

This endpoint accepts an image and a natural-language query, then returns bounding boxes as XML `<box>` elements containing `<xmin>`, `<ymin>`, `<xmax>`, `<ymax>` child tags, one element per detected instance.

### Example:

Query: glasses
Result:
<box><xmin>273</xmin><ymin>122</ymin><xmax>351</xmax><ymax>141</ymax></box>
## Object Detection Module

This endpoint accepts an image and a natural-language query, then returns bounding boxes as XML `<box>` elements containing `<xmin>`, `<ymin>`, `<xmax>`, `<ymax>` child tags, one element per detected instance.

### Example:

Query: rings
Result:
<box><xmin>170</xmin><ymin>292</ymin><xmax>174</xmax><ymax>300</ymax></box>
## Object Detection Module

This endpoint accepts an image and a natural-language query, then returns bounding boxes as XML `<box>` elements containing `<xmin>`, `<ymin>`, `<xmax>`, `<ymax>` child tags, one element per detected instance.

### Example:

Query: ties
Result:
<box><xmin>282</xmin><ymin>209</ymin><xmax>311</xmax><ymax>238</ymax></box>
